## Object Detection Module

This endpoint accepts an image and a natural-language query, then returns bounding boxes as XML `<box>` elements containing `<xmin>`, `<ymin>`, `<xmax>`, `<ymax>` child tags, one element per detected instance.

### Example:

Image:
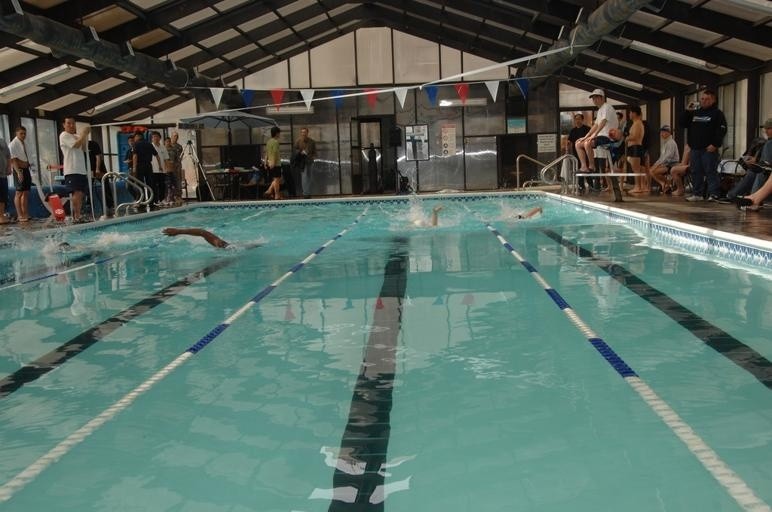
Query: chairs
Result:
<box><xmin>664</xmin><ymin>142</ymin><xmax>763</xmax><ymax>201</ymax></box>
<box><xmin>205</xmin><ymin>162</ymin><xmax>289</xmax><ymax>199</ymax></box>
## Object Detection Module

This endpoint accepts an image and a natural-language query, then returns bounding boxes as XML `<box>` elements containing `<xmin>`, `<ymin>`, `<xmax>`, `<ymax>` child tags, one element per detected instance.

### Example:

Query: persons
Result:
<box><xmin>124</xmin><ymin>130</ymin><xmax>183</xmax><ymax>209</ymax></box>
<box><xmin>579</xmin><ymin>266</ymin><xmax>624</xmax><ymax>325</ymax></box>
<box><xmin>409</xmin><ymin>205</ymin><xmax>445</xmax><ymax>227</ymax></box>
<box><xmin>58</xmin><ymin>116</ymin><xmax>92</xmax><ymax>222</ymax></box>
<box><xmin>0</xmin><ymin>136</ymin><xmax>12</xmax><ymax>226</ymax></box>
<box><xmin>262</xmin><ymin>128</ymin><xmax>285</xmax><ymax>200</ymax></box>
<box><xmin>84</xmin><ymin>139</ymin><xmax>115</xmax><ymax>215</ymax></box>
<box><xmin>567</xmin><ymin>87</ymin><xmax>772</xmax><ymax>213</ymax></box>
<box><xmin>161</xmin><ymin>226</ymin><xmax>244</xmax><ymax>255</ymax></box>
<box><xmin>291</xmin><ymin>127</ymin><xmax>318</xmax><ymax>199</ymax></box>
<box><xmin>8</xmin><ymin>126</ymin><xmax>38</xmax><ymax>222</ymax></box>
<box><xmin>511</xmin><ymin>205</ymin><xmax>543</xmax><ymax>219</ymax></box>
<box><xmin>70</xmin><ymin>263</ymin><xmax>97</xmax><ymax>327</ymax></box>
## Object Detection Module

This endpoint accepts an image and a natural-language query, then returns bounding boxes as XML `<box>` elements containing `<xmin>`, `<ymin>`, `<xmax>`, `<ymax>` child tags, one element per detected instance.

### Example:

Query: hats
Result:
<box><xmin>660</xmin><ymin>126</ymin><xmax>671</xmax><ymax>133</ymax></box>
<box><xmin>760</xmin><ymin>118</ymin><xmax>772</xmax><ymax>128</ymax></box>
<box><xmin>589</xmin><ymin>88</ymin><xmax>604</xmax><ymax>98</ymax></box>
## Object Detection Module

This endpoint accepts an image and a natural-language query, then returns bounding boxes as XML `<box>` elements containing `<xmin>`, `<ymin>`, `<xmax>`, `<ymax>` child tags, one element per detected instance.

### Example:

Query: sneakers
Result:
<box><xmin>685</xmin><ymin>193</ymin><xmax>758</xmax><ymax>211</ymax></box>
